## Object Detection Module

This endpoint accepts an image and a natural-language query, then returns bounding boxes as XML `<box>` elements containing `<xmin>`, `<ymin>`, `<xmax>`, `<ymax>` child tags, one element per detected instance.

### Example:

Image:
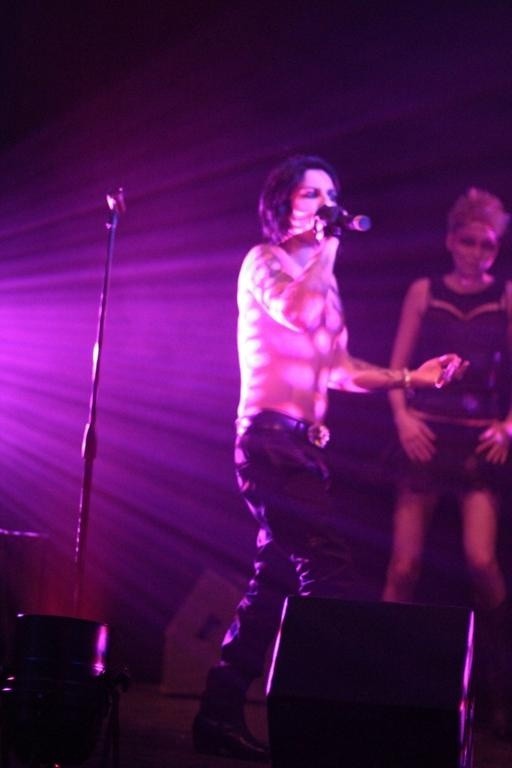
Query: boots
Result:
<box><xmin>191</xmin><ymin>667</ymin><xmax>267</xmax><ymax>759</ymax></box>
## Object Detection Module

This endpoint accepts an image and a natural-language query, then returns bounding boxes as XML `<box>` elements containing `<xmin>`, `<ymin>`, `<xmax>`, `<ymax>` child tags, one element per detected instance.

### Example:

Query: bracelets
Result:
<box><xmin>403</xmin><ymin>367</ymin><xmax>415</xmax><ymax>400</ymax></box>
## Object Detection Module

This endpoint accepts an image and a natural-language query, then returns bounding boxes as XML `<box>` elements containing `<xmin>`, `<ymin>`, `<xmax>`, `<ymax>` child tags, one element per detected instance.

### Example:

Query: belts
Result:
<box><xmin>246</xmin><ymin>413</ymin><xmax>330</xmax><ymax>449</ymax></box>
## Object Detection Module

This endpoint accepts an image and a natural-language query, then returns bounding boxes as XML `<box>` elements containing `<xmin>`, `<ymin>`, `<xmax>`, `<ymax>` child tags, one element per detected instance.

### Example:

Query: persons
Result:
<box><xmin>384</xmin><ymin>188</ymin><xmax>512</xmax><ymax>740</ymax></box>
<box><xmin>192</xmin><ymin>157</ymin><xmax>469</xmax><ymax>761</ymax></box>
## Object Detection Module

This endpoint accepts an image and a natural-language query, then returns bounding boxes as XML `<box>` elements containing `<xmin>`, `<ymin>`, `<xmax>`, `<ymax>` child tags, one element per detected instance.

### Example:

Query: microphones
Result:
<box><xmin>337</xmin><ymin>215</ymin><xmax>372</xmax><ymax>231</ymax></box>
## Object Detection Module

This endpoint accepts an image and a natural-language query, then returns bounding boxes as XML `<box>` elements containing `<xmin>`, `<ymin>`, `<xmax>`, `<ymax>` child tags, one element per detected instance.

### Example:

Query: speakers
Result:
<box><xmin>0</xmin><ymin>614</ymin><xmax>112</xmax><ymax>768</ymax></box>
<box><xmin>266</xmin><ymin>596</ymin><xmax>477</xmax><ymax>768</ymax></box>
